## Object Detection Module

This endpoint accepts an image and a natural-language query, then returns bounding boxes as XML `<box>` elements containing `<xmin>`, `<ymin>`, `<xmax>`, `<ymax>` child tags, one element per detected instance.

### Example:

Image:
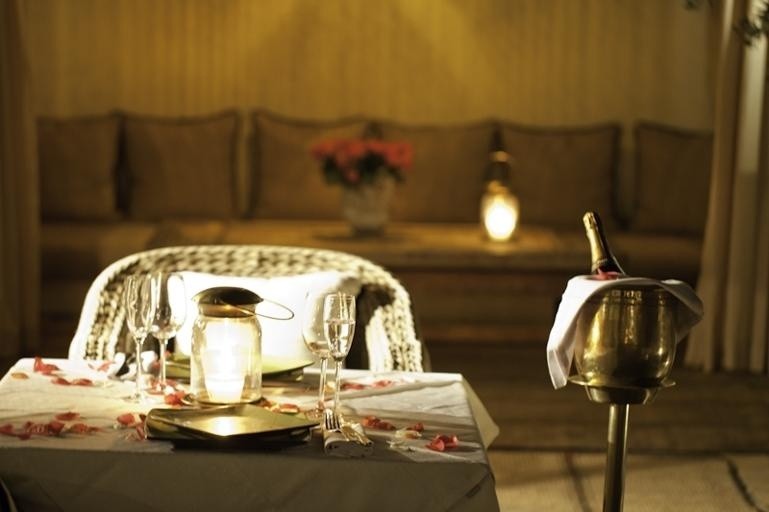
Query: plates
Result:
<box><xmin>148</xmin><ymin>401</ymin><xmax>321</xmax><ymax>450</ymax></box>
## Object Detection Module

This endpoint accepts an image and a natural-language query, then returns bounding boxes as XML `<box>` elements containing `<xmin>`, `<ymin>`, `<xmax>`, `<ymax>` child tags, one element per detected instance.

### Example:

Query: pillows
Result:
<box><xmin>119</xmin><ymin>109</ymin><xmax>241</xmax><ymax>222</ymax></box>
<box><xmin>377</xmin><ymin>119</ymin><xmax>494</xmax><ymax>224</ymax></box>
<box><xmin>251</xmin><ymin>109</ymin><xmax>369</xmax><ymax>224</ymax></box>
<box><xmin>35</xmin><ymin>113</ymin><xmax>121</xmax><ymax>226</ymax></box>
<box><xmin>168</xmin><ymin>266</ymin><xmax>364</xmax><ymax>371</ymax></box>
<box><xmin>631</xmin><ymin>120</ymin><xmax>715</xmax><ymax>234</ymax></box>
<box><xmin>498</xmin><ymin>122</ymin><xmax>622</xmax><ymax>230</ymax></box>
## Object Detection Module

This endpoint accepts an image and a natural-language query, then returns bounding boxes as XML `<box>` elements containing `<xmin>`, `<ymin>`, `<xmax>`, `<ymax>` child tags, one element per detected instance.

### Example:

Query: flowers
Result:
<box><xmin>308</xmin><ymin>137</ymin><xmax>415</xmax><ymax>184</ymax></box>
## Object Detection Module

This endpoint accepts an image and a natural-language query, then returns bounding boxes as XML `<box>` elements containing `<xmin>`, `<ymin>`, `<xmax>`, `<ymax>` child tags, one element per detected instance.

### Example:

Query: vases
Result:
<box><xmin>342</xmin><ymin>183</ymin><xmax>392</xmax><ymax>234</ymax></box>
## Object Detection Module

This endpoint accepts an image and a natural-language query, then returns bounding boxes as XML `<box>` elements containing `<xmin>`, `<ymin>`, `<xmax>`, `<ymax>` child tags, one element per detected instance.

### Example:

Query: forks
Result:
<box><xmin>324</xmin><ymin>413</ymin><xmax>356</xmax><ymax>449</ymax></box>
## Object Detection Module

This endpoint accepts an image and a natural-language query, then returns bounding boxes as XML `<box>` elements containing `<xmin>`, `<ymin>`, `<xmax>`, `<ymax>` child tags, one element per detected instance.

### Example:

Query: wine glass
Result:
<box><xmin>300</xmin><ymin>290</ymin><xmax>357</xmax><ymax>422</ymax></box>
<box><xmin>122</xmin><ymin>270</ymin><xmax>189</xmax><ymax>397</ymax></box>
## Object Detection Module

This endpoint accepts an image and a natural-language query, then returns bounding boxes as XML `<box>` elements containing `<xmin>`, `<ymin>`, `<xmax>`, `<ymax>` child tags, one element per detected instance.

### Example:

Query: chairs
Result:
<box><xmin>68</xmin><ymin>243</ymin><xmax>421</xmax><ymax>376</ymax></box>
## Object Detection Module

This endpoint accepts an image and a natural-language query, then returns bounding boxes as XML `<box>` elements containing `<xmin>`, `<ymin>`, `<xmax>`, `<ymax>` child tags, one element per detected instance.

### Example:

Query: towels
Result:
<box><xmin>546</xmin><ymin>274</ymin><xmax>706</xmax><ymax>390</ymax></box>
<box><xmin>324</xmin><ymin>428</ymin><xmax>373</xmax><ymax>458</ymax></box>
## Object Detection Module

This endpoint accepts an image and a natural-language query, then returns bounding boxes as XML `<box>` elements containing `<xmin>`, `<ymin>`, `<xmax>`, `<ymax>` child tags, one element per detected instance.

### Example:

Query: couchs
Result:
<box><xmin>39</xmin><ymin>213</ymin><xmax>705</xmax><ymax>341</ymax></box>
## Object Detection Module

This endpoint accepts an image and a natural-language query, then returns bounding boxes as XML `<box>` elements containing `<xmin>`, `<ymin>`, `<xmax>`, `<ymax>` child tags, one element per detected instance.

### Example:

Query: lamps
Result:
<box><xmin>481</xmin><ymin>134</ymin><xmax>519</xmax><ymax>242</ymax></box>
<box><xmin>182</xmin><ymin>286</ymin><xmax>295</xmax><ymax>407</ymax></box>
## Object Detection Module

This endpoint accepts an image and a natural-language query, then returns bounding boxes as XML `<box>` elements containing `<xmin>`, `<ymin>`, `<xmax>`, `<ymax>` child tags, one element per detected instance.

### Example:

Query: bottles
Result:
<box><xmin>582</xmin><ymin>208</ymin><xmax>628</xmax><ymax>280</ymax></box>
